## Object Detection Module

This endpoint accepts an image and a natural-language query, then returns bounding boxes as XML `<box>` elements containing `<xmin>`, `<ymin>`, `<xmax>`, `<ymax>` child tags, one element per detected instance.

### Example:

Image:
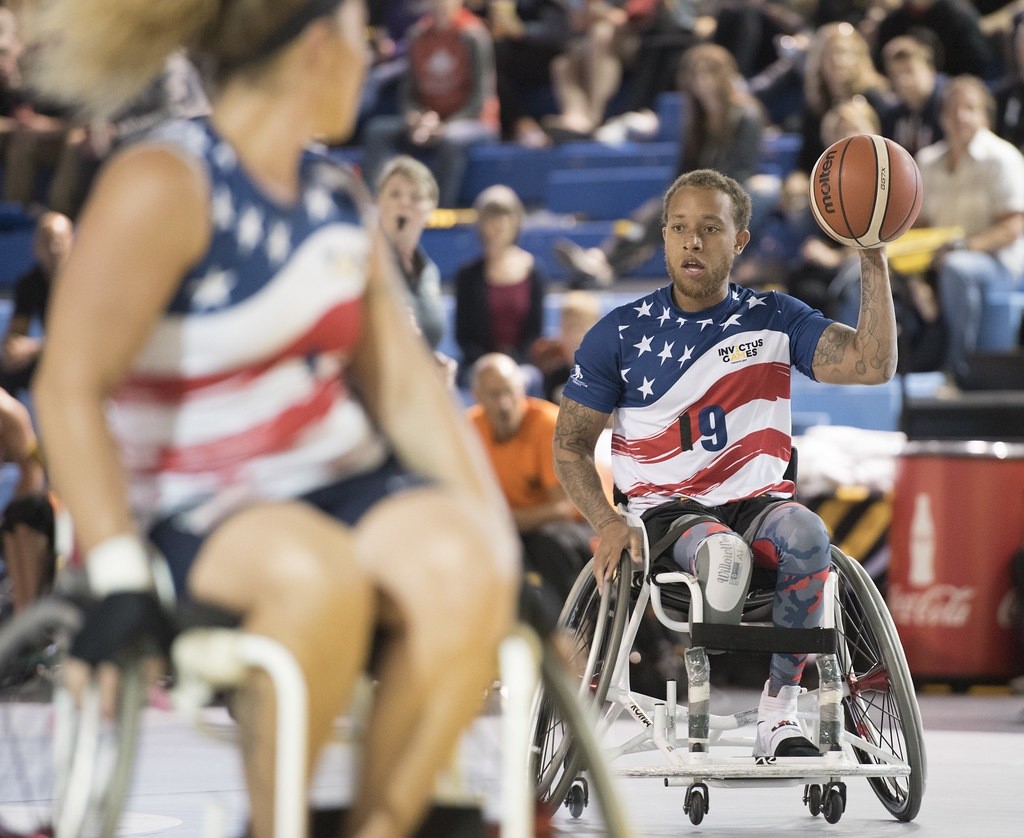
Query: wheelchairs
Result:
<box><xmin>2</xmin><ymin>556</ymin><xmax>636</xmax><ymax>838</ymax></box>
<box><xmin>531</xmin><ymin>486</ymin><xmax>928</xmax><ymax>829</ymax></box>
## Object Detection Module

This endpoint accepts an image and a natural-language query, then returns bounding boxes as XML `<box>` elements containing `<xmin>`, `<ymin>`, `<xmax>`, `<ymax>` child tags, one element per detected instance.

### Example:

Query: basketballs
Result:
<box><xmin>809</xmin><ymin>135</ymin><xmax>925</xmax><ymax>249</ymax></box>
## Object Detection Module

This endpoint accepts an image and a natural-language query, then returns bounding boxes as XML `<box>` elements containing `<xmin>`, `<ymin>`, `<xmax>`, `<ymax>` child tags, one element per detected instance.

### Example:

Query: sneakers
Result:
<box><xmin>751</xmin><ymin>678</ymin><xmax>824</xmax><ymax>757</ymax></box>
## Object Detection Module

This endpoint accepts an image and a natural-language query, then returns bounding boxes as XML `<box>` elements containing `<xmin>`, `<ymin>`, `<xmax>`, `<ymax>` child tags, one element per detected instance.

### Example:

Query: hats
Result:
<box><xmin>471</xmin><ymin>184</ymin><xmax>519</xmax><ymax>216</ymax></box>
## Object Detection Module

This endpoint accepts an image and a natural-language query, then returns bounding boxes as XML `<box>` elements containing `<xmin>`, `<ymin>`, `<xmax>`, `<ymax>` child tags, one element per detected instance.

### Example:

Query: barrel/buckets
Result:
<box><xmin>889</xmin><ymin>442</ymin><xmax>1024</xmax><ymax>680</ymax></box>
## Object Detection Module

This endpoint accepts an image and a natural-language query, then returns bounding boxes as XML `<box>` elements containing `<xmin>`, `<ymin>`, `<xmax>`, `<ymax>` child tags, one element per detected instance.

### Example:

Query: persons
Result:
<box><xmin>30</xmin><ymin>0</ymin><xmax>525</xmax><ymax>838</ymax></box>
<box><xmin>0</xmin><ymin>0</ymin><xmax>1023</xmax><ymax>626</ymax></box>
<box><xmin>551</xmin><ymin>170</ymin><xmax>901</xmax><ymax>759</ymax></box>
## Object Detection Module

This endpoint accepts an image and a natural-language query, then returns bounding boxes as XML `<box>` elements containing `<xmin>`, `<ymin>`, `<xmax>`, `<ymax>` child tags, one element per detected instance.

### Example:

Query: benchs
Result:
<box><xmin>0</xmin><ymin>1</ymin><xmax>1024</xmax><ymax>514</ymax></box>
